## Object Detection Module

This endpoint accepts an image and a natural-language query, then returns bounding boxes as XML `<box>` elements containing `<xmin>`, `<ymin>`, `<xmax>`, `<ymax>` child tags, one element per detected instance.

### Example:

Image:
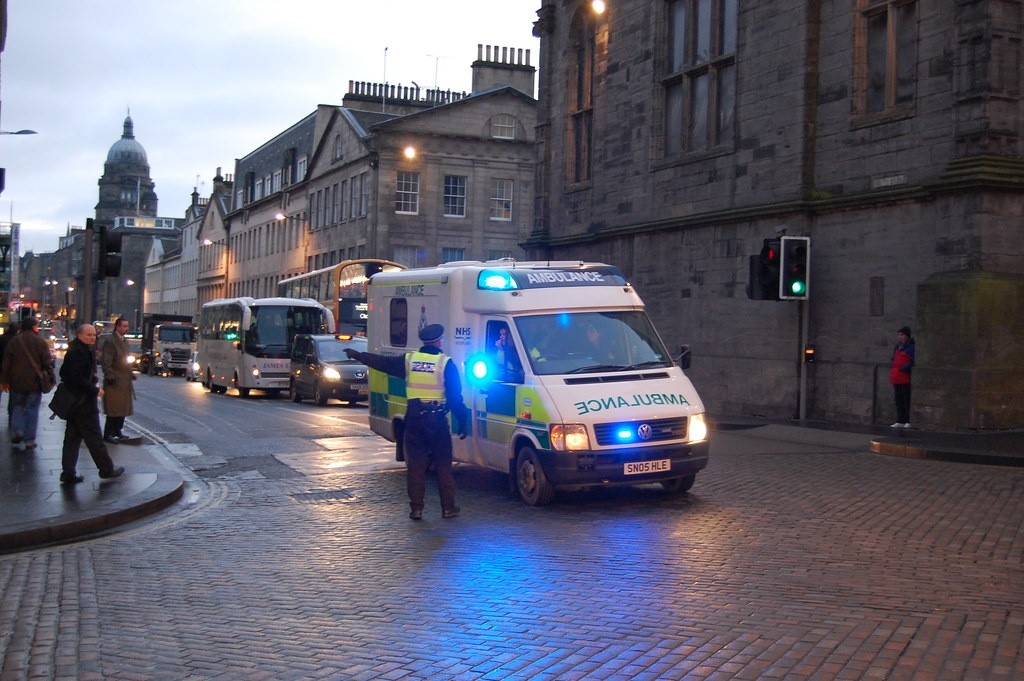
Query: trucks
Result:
<box><xmin>93</xmin><ymin>321</ymin><xmax>116</xmax><ymax>365</ymax></box>
<box><xmin>141</xmin><ymin>313</ymin><xmax>199</xmax><ymax>376</ymax></box>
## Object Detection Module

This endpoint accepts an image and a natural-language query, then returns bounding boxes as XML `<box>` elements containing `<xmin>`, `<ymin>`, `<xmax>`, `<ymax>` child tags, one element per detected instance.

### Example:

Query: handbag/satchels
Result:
<box><xmin>48</xmin><ymin>383</ymin><xmax>80</xmax><ymax>420</ymax></box>
<box><xmin>39</xmin><ymin>370</ymin><xmax>57</xmax><ymax>394</ymax></box>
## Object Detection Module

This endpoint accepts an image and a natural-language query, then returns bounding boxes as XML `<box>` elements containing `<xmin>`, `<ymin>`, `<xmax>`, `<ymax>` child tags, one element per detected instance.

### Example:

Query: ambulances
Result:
<box><xmin>365</xmin><ymin>258</ymin><xmax>709</xmax><ymax>507</ymax></box>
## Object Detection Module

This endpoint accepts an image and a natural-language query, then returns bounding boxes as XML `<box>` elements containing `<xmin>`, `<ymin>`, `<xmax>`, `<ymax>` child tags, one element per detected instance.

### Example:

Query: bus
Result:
<box><xmin>197</xmin><ymin>297</ymin><xmax>335</xmax><ymax>399</ymax></box>
<box><xmin>276</xmin><ymin>259</ymin><xmax>408</xmax><ymax>337</ymax></box>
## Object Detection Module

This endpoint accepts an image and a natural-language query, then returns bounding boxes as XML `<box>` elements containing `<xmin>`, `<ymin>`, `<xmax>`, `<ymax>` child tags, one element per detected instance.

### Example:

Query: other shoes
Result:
<box><xmin>903</xmin><ymin>422</ymin><xmax>912</xmax><ymax>429</ymax></box>
<box><xmin>442</xmin><ymin>506</ymin><xmax>460</xmax><ymax>518</ymax></box>
<box><xmin>99</xmin><ymin>466</ymin><xmax>125</xmax><ymax>478</ymax></box>
<box><xmin>11</xmin><ymin>431</ymin><xmax>26</xmax><ymax>443</ymax></box>
<box><xmin>890</xmin><ymin>423</ymin><xmax>904</xmax><ymax>428</ymax></box>
<box><xmin>60</xmin><ymin>472</ymin><xmax>84</xmax><ymax>483</ymax></box>
<box><xmin>409</xmin><ymin>510</ymin><xmax>421</xmax><ymax>519</ymax></box>
<box><xmin>25</xmin><ymin>443</ymin><xmax>37</xmax><ymax>450</ymax></box>
<box><xmin>117</xmin><ymin>434</ymin><xmax>129</xmax><ymax>439</ymax></box>
<box><xmin>105</xmin><ymin>437</ymin><xmax>119</xmax><ymax>444</ymax></box>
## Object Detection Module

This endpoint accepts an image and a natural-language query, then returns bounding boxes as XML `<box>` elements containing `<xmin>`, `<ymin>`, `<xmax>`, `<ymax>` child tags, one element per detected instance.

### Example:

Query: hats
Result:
<box><xmin>418</xmin><ymin>324</ymin><xmax>444</xmax><ymax>344</ymax></box>
<box><xmin>898</xmin><ymin>327</ymin><xmax>911</xmax><ymax>337</ymax></box>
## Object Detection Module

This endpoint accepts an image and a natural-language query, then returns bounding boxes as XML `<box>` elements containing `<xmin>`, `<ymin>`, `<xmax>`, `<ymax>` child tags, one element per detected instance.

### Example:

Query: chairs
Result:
<box><xmin>340</xmin><ymin>280</ymin><xmax>369</xmax><ymax>298</ymax></box>
<box><xmin>300</xmin><ymin>325</ymin><xmax>311</xmax><ymax>334</ymax></box>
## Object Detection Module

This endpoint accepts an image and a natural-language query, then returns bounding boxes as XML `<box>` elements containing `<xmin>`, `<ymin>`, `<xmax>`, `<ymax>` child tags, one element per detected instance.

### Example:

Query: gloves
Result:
<box><xmin>458</xmin><ymin>420</ymin><xmax>468</xmax><ymax>440</ymax></box>
<box><xmin>342</xmin><ymin>348</ymin><xmax>360</xmax><ymax>359</ymax></box>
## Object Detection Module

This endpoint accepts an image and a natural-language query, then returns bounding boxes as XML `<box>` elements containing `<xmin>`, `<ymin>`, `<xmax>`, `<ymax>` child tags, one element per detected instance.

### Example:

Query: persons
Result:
<box><xmin>584</xmin><ymin>322</ymin><xmax>617</xmax><ymax>364</ymax></box>
<box><xmin>58</xmin><ymin>324</ymin><xmax>126</xmax><ymax>482</ymax></box>
<box><xmin>494</xmin><ymin>326</ymin><xmax>546</xmax><ymax>371</ymax></box>
<box><xmin>101</xmin><ymin>317</ymin><xmax>137</xmax><ymax>444</ymax></box>
<box><xmin>161</xmin><ymin>349</ymin><xmax>172</xmax><ymax>373</ymax></box>
<box><xmin>0</xmin><ymin>317</ymin><xmax>51</xmax><ymax>449</ymax></box>
<box><xmin>890</xmin><ymin>327</ymin><xmax>914</xmax><ymax>429</ymax></box>
<box><xmin>342</xmin><ymin>324</ymin><xmax>468</xmax><ymax>520</ymax></box>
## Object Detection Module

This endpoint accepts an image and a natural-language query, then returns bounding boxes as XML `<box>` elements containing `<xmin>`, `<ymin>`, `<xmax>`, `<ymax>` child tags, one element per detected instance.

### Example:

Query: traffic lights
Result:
<box><xmin>747</xmin><ymin>239</ymin><xmax>780</xmax><ymax>302</ymax></box>
<box><xmin>779</xmin><ymin>235</ymin><xmax>809</xmax><ymax>301</ymax></box>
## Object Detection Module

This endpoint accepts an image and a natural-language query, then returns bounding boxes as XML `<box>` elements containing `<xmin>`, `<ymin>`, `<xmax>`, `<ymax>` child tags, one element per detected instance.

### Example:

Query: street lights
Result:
<box><xmin>127</xmin><ymin>279</ymin><xmax>138</xmax><ymax>331</ymax></box>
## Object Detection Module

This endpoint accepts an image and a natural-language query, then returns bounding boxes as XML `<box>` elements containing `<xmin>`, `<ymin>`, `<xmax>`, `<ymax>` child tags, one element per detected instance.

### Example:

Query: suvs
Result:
<box><xmin>289</xmin><ymin>334</ymin><xmax>368</xmax><ymax>407</ymax></box>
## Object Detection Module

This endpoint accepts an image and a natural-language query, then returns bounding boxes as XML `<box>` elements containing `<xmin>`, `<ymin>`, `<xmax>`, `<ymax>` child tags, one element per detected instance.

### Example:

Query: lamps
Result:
<box><xmin>204</xmin><ymin>239</ymin><xmax>228</xmax><ymax>245</ymax></box>
<box><xmin>276</xmin><ymin>212</ymin><xmax>308</xmax><ymax>221</ymax></box>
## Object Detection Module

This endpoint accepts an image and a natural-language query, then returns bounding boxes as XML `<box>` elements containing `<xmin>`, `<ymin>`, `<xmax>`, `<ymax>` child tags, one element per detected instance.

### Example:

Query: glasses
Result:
<box><xmin>897</xmin><ymin>334</ymin><xmax>904</xmax><ymax>336</ymax></box>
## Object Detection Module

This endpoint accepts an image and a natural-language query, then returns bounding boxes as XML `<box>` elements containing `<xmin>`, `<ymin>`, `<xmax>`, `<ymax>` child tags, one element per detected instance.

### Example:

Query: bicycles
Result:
<box><xmin>155</xmin><ymin>359</ymin><xmax>171</xmax><ymax>378</ymax></box>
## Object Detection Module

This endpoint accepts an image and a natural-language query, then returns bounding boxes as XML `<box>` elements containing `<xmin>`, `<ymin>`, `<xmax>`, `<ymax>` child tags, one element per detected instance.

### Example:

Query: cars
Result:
<box><xmin>54</xmin><ymin>338</ymin><xmax>69</xmax><ymax>350</ymax></box>
<box><xmin>185</xmin><ymin>352</ymin><xmax>203</xmax><ymax>382</ymax></box>
<box><xmin>34</xmin><ymin>328</ymin><xmax>55</xmax><ymax>349</ymax></box>
<box><xmin>126</xmin><ymin>339</ymin><xmax>142</xmax><ymax>371</ymax></box>
